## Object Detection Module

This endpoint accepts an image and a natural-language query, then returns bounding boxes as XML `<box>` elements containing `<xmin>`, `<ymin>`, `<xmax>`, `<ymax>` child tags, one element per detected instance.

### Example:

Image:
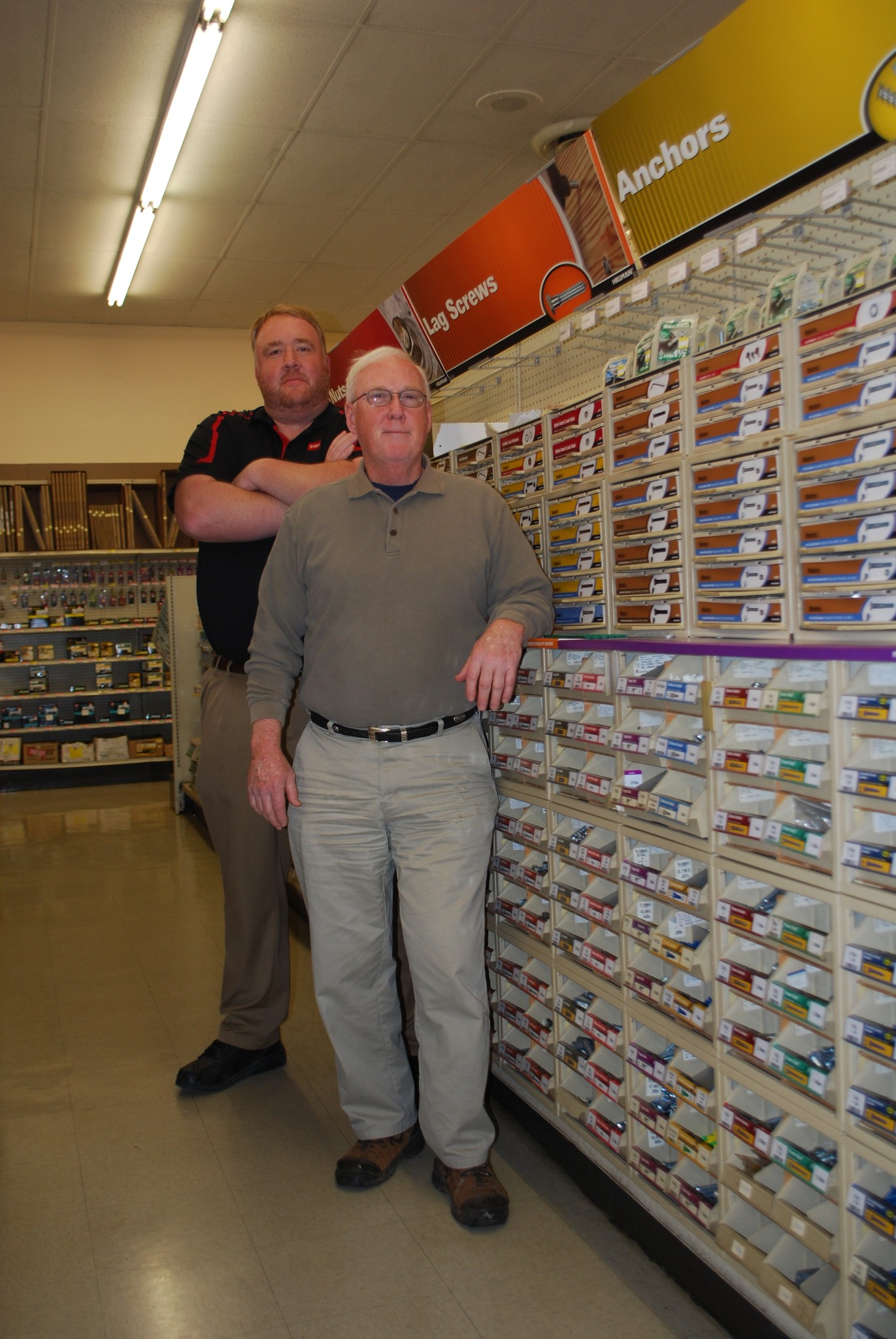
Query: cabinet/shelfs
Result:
<box><xmin>0</xmin><ymin>549</ymin><xmax>198</xmax><ymax>771</ymax></box>
<box><xmin>483</xmin><ymin>644</ymin><xmax>896</xmax><ymax>1339</ymax></box>
<box><xmin>432</xmin><ymin>283</ymin><xmax>896</xmax><ymax>629</ymax></box>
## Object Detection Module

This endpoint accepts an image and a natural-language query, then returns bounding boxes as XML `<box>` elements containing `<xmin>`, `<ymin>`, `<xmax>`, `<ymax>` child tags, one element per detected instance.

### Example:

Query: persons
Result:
<box><xmin>244</xmin><ymin>346</ymin><xmax>553</xmax><ymax>1227</ymax></box>
<box><xmin>169</xmin><ymin>305</ymin><xmax>364</xmax><ymax>1096</ymax></box>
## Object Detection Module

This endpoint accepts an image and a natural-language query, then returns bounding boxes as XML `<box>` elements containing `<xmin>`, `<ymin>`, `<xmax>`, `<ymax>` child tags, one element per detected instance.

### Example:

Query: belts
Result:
<box><xmin>310</xmin><ymin>705</ymin><xmax>477</xmax><ymax>743</ymax></box>
<box><xmin>212</xmin><ymin>655</ymin><xmax>246</xmax><ymax>674</ymax></box>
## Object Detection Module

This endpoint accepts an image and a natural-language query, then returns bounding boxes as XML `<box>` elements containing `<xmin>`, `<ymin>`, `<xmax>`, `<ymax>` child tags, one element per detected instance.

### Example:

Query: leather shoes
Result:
<box><xmin>175</xmin><ymin>1039</ymin><xmax>286</xmax><ymax>1092</ymax></box>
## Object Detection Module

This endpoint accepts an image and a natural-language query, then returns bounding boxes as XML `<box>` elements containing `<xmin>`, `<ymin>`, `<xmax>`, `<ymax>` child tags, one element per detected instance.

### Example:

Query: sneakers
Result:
<box><xmin>431</xmin><ymin>1156</ymin><xmax>509</xmax><ymax>1226</ymax></box>
<box><xmin>335</xmin><ymin>1120</ymin><xmax>425</xmax><ymax>1187</ymax></box>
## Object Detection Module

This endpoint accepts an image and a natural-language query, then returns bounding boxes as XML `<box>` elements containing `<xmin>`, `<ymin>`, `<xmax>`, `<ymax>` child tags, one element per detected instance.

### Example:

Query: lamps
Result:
<box><xmin>106</xmin><ymin>0</ymin><xmax>236</xmax><ymax>306</ymax></box>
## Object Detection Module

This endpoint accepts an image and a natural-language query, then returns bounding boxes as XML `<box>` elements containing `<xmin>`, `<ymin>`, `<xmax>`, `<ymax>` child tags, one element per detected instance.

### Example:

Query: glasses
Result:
<box><xmin>351</xmin><ymin>389</ymin><xmax>427</xmax><ymax>408</ymax></box>
